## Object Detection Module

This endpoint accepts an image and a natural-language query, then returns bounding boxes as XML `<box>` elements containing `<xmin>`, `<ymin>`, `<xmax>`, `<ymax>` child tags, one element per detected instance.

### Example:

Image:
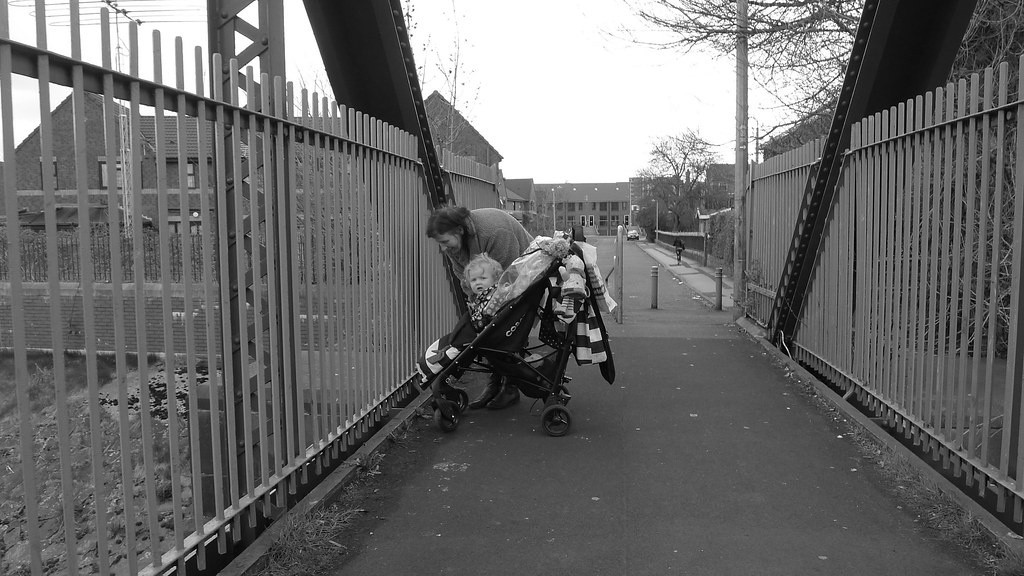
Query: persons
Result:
<box><xmin>459</xmin><ymin>251</ymin><xmax>504</xmax><ymax>300</ymax></box>
<box><xmin>425</xmin><ymin>204</ymin><xmax>543</xmax><ymax>406</ymax></box>
<box><xmin>673</xmin><ymin>235</ymin><xmax>685</xmax><ymax>265</ymax></box>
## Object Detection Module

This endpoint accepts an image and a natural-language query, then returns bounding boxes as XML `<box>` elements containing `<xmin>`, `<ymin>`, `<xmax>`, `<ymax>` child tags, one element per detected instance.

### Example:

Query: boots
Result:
<box><xmin>487</xmin><ymin>375</ymin><xmax>520</xmax><ymax>409</ymax></box>
<box><xmin>469</xmin><ymin>374</ymin><xmax>501</xmax><ymax>409</ymax></box>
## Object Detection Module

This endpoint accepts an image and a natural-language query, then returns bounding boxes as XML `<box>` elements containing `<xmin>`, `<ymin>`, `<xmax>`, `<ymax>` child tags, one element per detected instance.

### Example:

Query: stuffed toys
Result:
<box><xmin>533</xmin><ymin>234</ymin><xmax>585</xmax><ymax>323</ymax></box>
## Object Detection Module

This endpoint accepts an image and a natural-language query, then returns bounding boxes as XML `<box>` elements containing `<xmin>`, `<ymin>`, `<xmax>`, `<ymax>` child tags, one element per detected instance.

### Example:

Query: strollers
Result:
<box><xmin>428</xmin><ymin>232</ymin><xmax>590</xmax><ymax>438</ymax></box>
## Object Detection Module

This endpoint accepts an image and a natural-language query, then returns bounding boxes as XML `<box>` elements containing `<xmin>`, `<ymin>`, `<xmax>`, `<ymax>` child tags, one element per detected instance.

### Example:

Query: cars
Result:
<box><xmin>627</xmin><ymin>230</ymin><xmax>640</xmax><ymax>240</ymax></box>
